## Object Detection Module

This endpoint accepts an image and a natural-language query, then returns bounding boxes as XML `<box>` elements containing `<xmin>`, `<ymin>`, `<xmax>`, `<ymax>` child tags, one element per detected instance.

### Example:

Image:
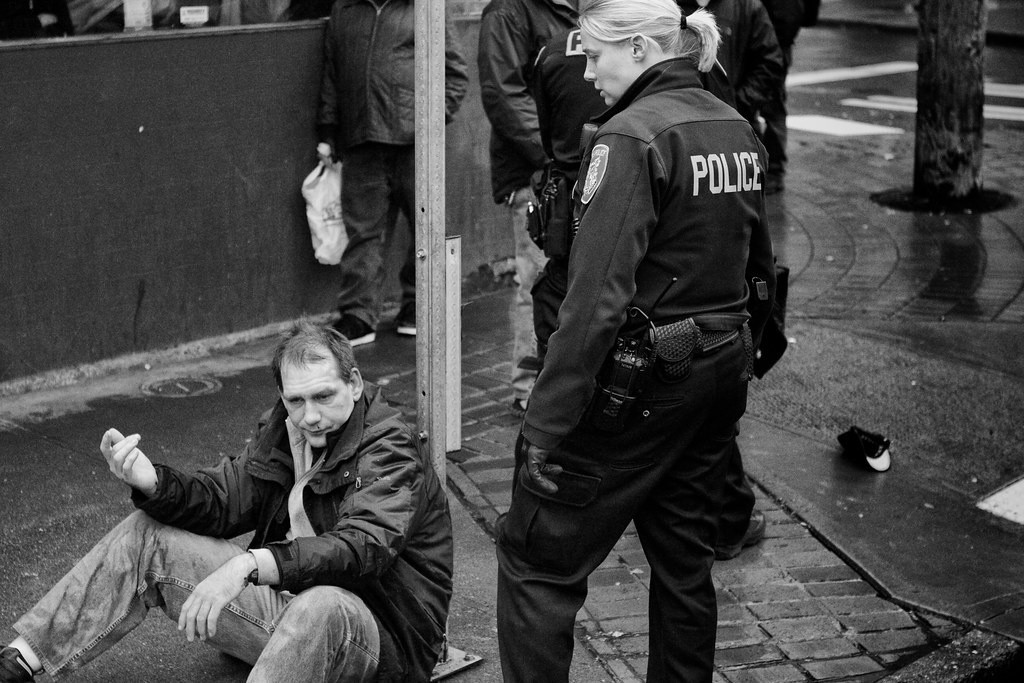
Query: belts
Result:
<box><xmin>695</xmin><ymin>329</ymin><xmax>740</xmax><ymax>353</ymax></box>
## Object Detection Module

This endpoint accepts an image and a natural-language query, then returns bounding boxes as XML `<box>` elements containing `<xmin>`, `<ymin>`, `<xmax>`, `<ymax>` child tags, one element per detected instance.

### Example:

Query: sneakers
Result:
<box><xmin>327</xmin><ymin>313</ymin><xmax>376</xmax><ymax>346</ymax></box>
<box><xmin>395</xmin><ymin>298</ymin><xmax>416</xmax><ymax>335</ymax></box>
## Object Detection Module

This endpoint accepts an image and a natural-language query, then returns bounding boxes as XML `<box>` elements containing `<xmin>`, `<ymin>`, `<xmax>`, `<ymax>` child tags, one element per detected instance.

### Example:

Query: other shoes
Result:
<box><xmin>766</xmin><ymin>175</ymin><xmax>785</xmax><ymax>194</ymax></box>
<box><xmin>511</xmin><ymin>398</ymin><xmax>528</xmax><ymax>418</ymax></box>
<box><xmin>0</xmin><ymin>646</ymin><xmax>45</xmax><ymax>683</ymax></box>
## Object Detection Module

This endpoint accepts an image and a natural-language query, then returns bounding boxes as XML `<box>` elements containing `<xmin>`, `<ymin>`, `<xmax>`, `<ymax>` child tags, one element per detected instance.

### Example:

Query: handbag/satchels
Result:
<box><xmin>301</xmin><ymin>155</ymin><xmax>350</xmax><ymax>264</ymax></box>
<box><xmin>526</xmin><ymin>166</ymin><xmax>571</xmax><ymax>261</ymax></box>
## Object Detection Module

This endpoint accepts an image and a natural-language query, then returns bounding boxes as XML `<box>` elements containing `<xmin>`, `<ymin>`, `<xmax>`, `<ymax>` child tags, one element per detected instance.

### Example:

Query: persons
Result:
<box><xmin>479</xmin><ymin>0</ymin><xmax>821</xmax><ymax>683</ymax></box>
<box><xmin>0</xmin><ymin>321</ymin><xmax>454</xmax><ymax>683</ymax></box>
<box><xmin>316</xmin><ymin>1</ymin><xmax>470</xmax><ymax>347</ymax></box>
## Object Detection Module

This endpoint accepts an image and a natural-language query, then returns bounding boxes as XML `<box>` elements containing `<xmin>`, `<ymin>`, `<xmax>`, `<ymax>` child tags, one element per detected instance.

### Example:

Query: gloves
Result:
<box><xmin>521</xmin><ymin>441</ymin><xmax>563</xmax><ymax>494</ymax></box>
